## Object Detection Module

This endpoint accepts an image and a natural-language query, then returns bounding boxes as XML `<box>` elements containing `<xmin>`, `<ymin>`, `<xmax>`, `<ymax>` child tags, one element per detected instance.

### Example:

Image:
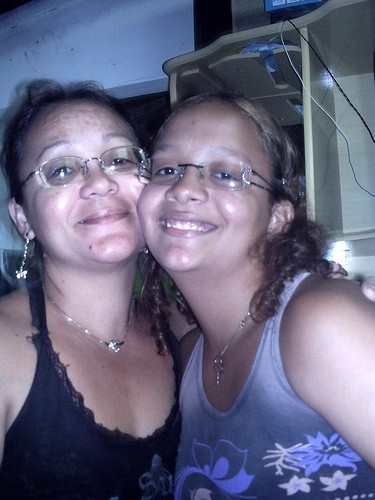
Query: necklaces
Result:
<box><xmin>44</xmin><ymin>287</ymin><xmax>161</xmax><ymax>356</ymax></box>
<box><xmin>203</xmin><ymin>309</ymin><xmax>259</xmax><ymax>388</ymax></box>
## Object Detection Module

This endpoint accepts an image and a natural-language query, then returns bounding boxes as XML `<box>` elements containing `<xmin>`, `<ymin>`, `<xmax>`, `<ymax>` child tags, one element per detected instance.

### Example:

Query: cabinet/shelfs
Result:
<box><xmin>161</xmin><ymin>0</ymin><xmax>375</xmax><ymax>284</ymax></box>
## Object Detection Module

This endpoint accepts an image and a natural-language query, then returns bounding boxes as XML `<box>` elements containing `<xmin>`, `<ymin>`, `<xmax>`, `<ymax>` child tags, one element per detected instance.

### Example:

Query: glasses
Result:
<box><xmin>138</xmin><ymin>152</ymin><xmax>284</xmax><ymax>197</ymax></box>
<box><xmin>15</xmin><ymin>145</ymin><xmax>147</xmax><ymax>194</ymax></box>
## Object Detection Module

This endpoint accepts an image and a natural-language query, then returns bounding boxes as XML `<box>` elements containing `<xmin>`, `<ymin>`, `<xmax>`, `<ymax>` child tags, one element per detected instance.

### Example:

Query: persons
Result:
<box><xmin>0</xmin><ymin>80</ymin><xmax>375</xmax><ymax>500</ymax></box>
<box><xmin>137</xmin><ymin>89</ymin><xmax>375</xmax><ymax>500</ymax></box>
